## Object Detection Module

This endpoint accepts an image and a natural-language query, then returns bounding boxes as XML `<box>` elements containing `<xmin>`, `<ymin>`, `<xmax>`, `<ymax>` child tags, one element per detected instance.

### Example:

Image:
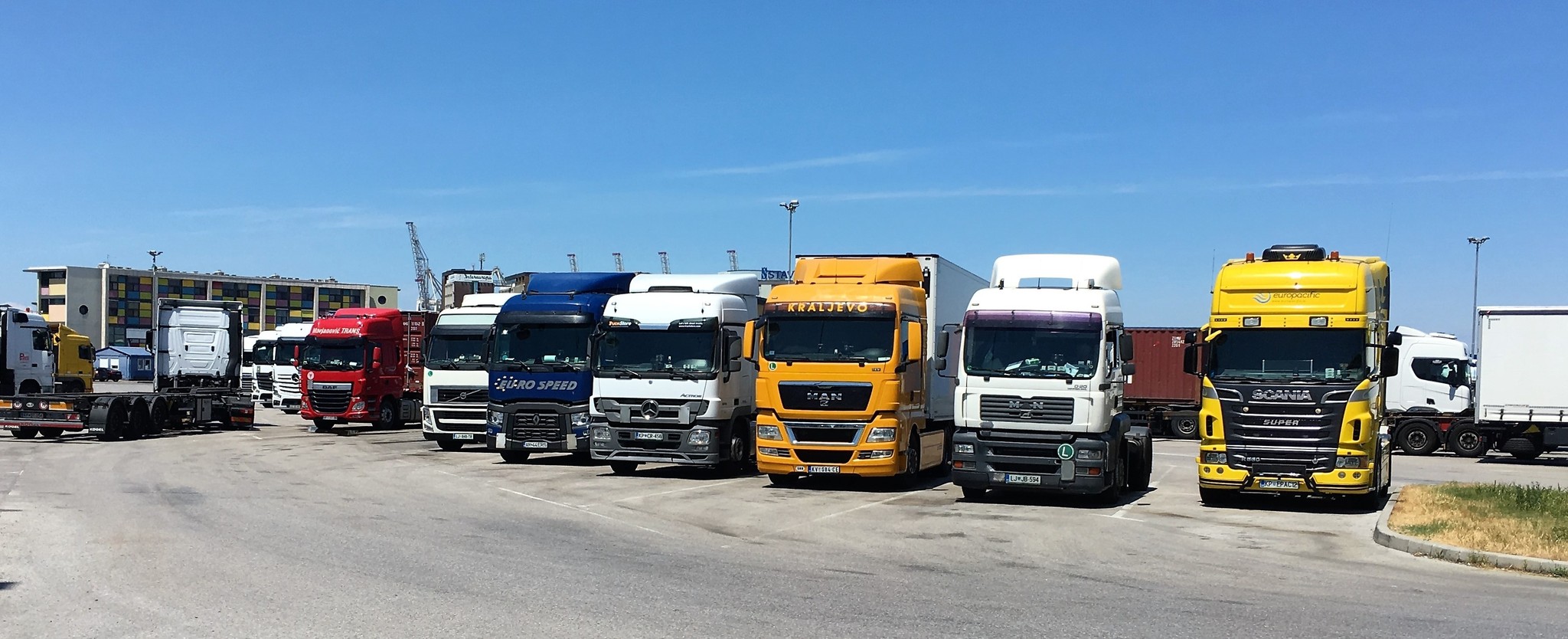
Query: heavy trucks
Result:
<box><xmin>0</xmin><ymin>296</ymin><xmax>255</xmax><ymax>443</ymax></box>
<box><xmin>1125</xmin><ymin>324</ymin><xmax>1476</xmax><ymax>441</ymax></box>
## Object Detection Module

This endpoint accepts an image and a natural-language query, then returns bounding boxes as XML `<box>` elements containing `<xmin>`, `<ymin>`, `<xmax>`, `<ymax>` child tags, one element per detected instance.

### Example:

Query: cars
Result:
<box><xmin>93</xmin><ymin>367</ymin><xmax>122</xmax><ymax>382</ymax></box>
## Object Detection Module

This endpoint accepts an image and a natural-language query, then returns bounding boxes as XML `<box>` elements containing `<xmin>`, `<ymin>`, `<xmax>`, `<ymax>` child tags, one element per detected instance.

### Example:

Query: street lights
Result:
<box><xmin>779</xmin><ymin>200</ymin><xmax>800</xmax><ymax>284</ymax></box>
<box><xmin>1466</xmin><ymin>236</ymin><xmax>1491</xmax><ymax>359</ymax></box>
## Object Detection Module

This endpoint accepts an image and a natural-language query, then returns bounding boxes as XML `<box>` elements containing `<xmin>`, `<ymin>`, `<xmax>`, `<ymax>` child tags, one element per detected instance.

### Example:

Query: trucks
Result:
<box><xmin>1381</xmin><ymin>305</ymin><xmax>1568</xmax><ymax>461</ymax></box>
<box><xmin>742</xmin><ymin>251</ymin><xmax>990</xmax><ymax>491</ymax></box>
<box><xmin>1183</xmin><ymin>245</ymin><xmax>1402</xmax><ymax>511</ymax></box>
<box><xmin>587</xmin><ymin>273</ymin><xmax>767</xmax><ymax>478</ymax></box>
<box><xmin>240</xmin><ymin>320</ymin><xmax>314</xmax><ymax>415</ymax></box>
<box><xmin>292</xmin><ymin>307</ymin><xmax>440</xmax><ymax>431</ymax></box>
<box><xmin>479</xmin><ymin>272</ymin><xmax>640</xmax><ymax>466</ymax></box>
<box><xmin>0</xmin><ymin>305</ymin><xmax>96</xmax><ymax>398</ymax></box>
<box><xmin>419</xmin><ymin>292</ymin><xmax>526</xmax><ymax>452</ymax></box>
<box><xmin>936</xmin><ymin>251</ymin><xmax>1153</xmax><ymax>504</ymax></box>
